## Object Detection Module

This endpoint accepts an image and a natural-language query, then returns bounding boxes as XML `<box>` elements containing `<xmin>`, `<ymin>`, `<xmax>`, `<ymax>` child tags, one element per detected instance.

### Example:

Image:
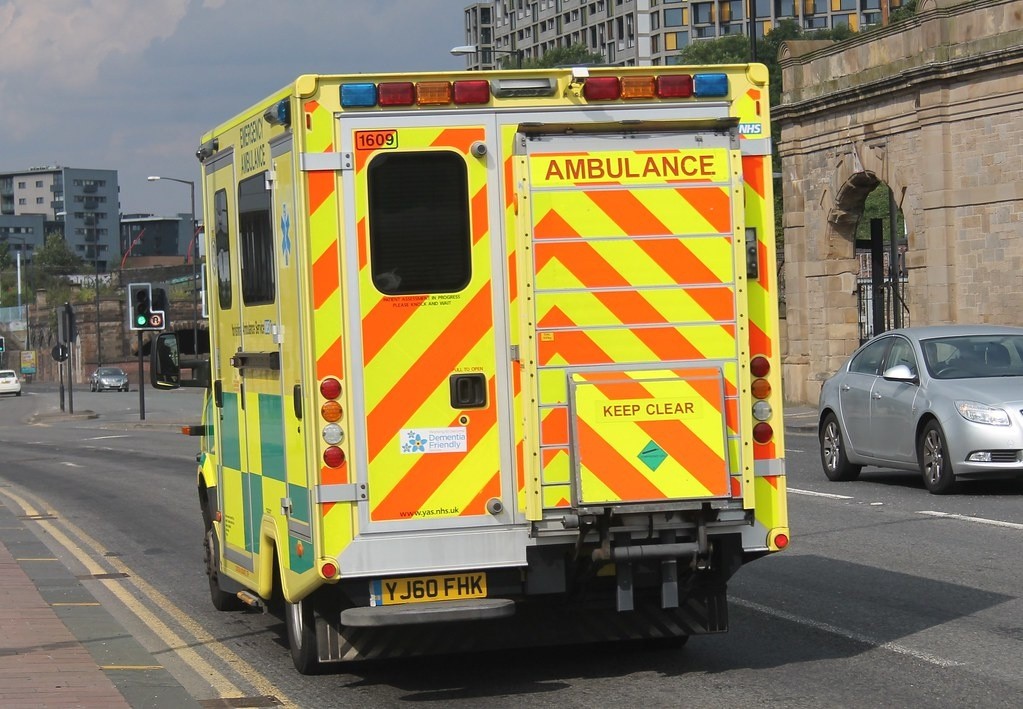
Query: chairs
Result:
<box><xmin>983</xmin><ymin>342</ymin><xmax>1011</xmax><ymax>369</ymax></box>
<box><xmin>903</xmin><ymin>343</ymin><xmax>939</xmax><ymax>372</ymax></box>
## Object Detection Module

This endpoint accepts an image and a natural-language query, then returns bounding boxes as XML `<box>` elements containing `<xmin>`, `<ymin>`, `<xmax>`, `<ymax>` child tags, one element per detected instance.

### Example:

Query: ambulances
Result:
<box><xmin>150</xmin><ymin>63</ymin><xmax>789</xmax><ymax>675</ymax></box>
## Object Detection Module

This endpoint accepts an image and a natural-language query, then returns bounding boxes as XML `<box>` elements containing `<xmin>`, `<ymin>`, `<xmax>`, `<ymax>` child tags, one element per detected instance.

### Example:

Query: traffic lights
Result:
<box><xmin>0</xmin><ymin>337</ymin><xmax>5</xmax><ymax>352</ymax></box>
<box><xmin>129</xmin><ymin>282</ymin><xmax>165</xmax><ymax>331</ymax></box>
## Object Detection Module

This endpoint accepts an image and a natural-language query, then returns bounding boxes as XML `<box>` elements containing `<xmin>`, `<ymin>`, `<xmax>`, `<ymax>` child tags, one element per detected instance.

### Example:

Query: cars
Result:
<box><xmin>818</xmin><ymin>323</ymin><xmax>1023</xmax><ymax>495</ymax></box>
<box><xmin>0</xmin><ymin>370</ymin><xmax>21</xmax><ymax>396</ymax></box>
<box><xmin>90</xmin><ymin>367</ymin><xmax>129</xmax><ymax>392</ymax></box>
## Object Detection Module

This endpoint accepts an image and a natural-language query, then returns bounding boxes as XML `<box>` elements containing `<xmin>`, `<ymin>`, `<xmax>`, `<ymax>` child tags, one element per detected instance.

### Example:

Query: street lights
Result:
<box><xmin>147</xmin><ymin>176</ymin><xmax>198</xmax><ymax>360</ymax></box>
<box><xmin>450</xmin><ymin>45</ymin><xmax>524</xmax><ymax>69</ymax></box>
